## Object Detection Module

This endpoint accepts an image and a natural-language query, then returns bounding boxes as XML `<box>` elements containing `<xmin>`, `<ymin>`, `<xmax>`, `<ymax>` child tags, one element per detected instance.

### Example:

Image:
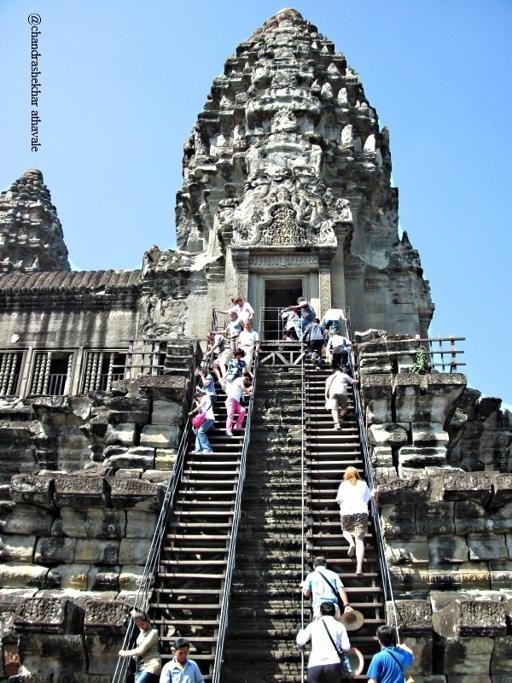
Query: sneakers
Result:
<box><xmin>312</xmin><ymin>353</ymin><xmax>316</xmax><ymax>363</ymax></box>
<box><xmin>348</xmin><ymin>544</ymin><xmax>355</xmax><ymax>557</ymax></box>
<box><xmin>333</xmin><ymin>415</ymin><xmax>344</xmax><ymax>430</ymax></box>
<box><xmin>356</xmin><ymin>572</ymin><xmax>363</xmax><ymax>575</ymax></box>
<box><xmin>315</xmin><ymin>366</ymin><xmax>320</xmax><ymax>371</ymax></box>
<box><xmin>226</xmin><ymin>425</ymin><xmax>244</xmax><ymax>435</ymax></box>
<box><xmin>189</xmin><ymin>450</ymin><xmax>213</xmax><ymax>455</ymax></box>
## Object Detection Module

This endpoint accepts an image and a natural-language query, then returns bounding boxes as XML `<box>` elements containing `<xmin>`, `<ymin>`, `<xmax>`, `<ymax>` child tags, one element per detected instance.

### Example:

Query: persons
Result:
<box><xmin>366</xmin><ymin>625</ymin><xmax>415</xmax><ymax>683</ymax></box>
<box><xmin>187</xmin><ymin>296</ymin><xmax>360</xmax><ymax>454</ymax></box>
<box><xmin>296</xmin><ymin>602</ymin><xmax>351</xmax><ymax>683</ymax></box>
<box><xmin>335</xmin><ymin>466</ymin><xmax>377</xmax><ymax>576</ymax></box>
<box><xmin>159</xmin><ymin>638</ymin><xmax>205</xmax><ymax>683</ymax></box>
<box><xmin>299</xmin><ymin>556</ymin><xmax>352</xmax><ymax>620</ymax></box>
<box><xmin>117</xmin><ymin>609</ymin><xmax>162</xmax><ymax>683</ymax></box>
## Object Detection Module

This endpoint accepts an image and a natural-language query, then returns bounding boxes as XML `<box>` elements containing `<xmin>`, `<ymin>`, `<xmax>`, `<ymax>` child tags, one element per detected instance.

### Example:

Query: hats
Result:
<box><xmin>338</xmin><ymin>608</ymin><xmax>365</xmax><ymax>632</ymax></box>
<box><xmin>192</xmin><ymin>425</ymin><xmax>198</xmax><ymax>435</ymax></box>
<box><xmin>403</xmin><ymin>670</ymin><xmax>424</xmax><ymax>683</ymax></box>
<box><xmin>348</xmin><ymin>648</ymin><xmax>365</xmax><ymax>677</ymax></box>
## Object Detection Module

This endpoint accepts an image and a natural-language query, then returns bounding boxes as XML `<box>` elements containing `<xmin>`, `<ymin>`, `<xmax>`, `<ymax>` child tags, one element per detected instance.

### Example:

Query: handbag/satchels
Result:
<box><xmin>336</xmin><ymin>592</ymin><xmax>344</xmax><ymax>615</ymax></box>
<box><xmin>340</xmin><ymin>653</ymin><xmax>352</xmax><ymax>679</ymax></box>
<box><xmin>192</xmin><ymin>414</ymin><xmax>205</xmax><ymax>427</ymax></box>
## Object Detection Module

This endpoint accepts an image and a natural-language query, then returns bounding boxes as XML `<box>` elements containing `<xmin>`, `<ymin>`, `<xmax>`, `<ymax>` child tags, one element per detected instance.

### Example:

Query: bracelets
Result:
<box><xmin>343</xmin><ymin>603</ymin><xmax>349</xmax><ymax>608</ymax></box>
